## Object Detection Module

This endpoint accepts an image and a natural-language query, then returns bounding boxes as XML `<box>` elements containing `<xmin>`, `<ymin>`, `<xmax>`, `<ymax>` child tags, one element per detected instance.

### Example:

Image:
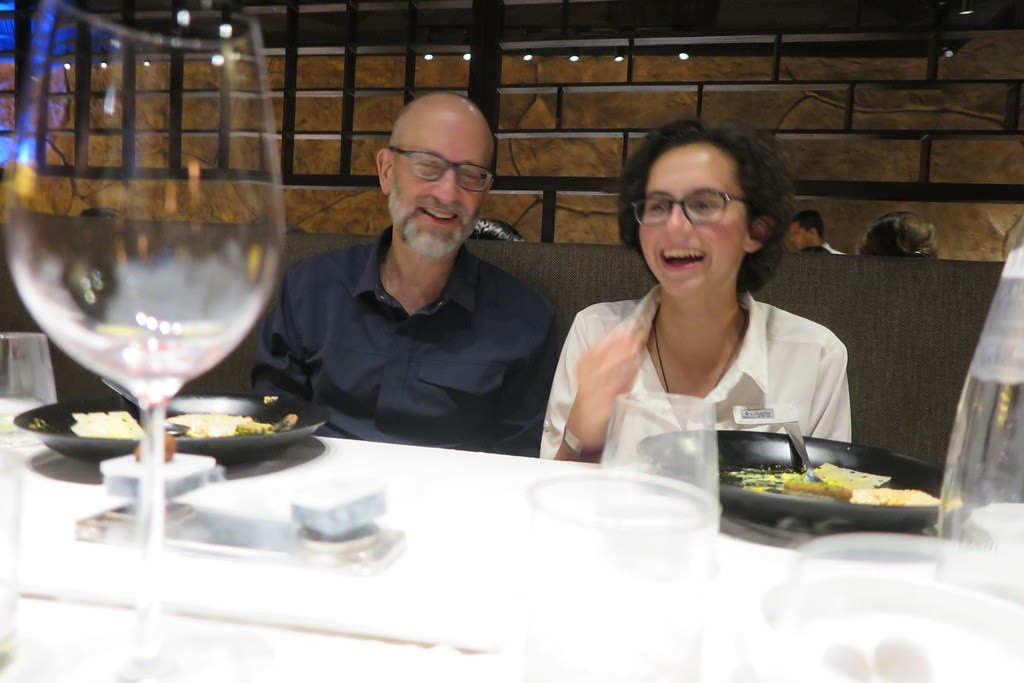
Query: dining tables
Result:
<box><xmin>0</xmin><ymin>398</ymin><xmax>1023</xmax><ymax>683</ymax></box>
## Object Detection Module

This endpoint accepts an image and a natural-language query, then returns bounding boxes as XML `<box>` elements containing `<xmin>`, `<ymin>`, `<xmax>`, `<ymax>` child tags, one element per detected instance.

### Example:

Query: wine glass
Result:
<box><xmin>10</xmin><ymin>0</ymin><xmax>283</xmax><ymax>683</ymax></box>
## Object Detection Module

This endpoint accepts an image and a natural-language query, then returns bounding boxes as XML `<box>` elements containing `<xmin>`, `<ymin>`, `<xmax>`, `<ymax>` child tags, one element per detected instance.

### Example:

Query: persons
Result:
<box><xmin>790</xmin><ymin>210</ymin><xmax>847</xmax><ymax>255</ymax></box>
<box><xmin>855</xmin><ymin>211</ymin><xmax>938</xmax><ymax>259</ymax></box>
<box><xmin>539</xmin><ymin>118</ymin><xmax>851</xmax><ymax>497</ymax></box>
<box><xmin>252</xmin><ymin>93</ymin><xmax>559</xmax><ymax>458</ymax></box>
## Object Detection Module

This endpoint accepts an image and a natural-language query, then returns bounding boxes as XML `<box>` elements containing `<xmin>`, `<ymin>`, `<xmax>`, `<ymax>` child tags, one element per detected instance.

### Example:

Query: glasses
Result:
<box><xmin>390</xmin><ymin>144</ymin><xmax>496</xmax><ymax>193</ymax></box>
<box><xmin>630</xmin><ymin>188</ymin><xmax>747</xmax><ymax>228</ymax></box>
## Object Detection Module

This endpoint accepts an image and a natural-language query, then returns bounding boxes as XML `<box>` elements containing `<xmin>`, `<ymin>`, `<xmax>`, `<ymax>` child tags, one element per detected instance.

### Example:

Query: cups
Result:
<box><xmin>0</xmin><ymin>334</ymin><xmax>57</xmax><ymax>445</ymax></box>
<box><xmin>755</xmin><ymin>540</ymin><xmax>1024</xmax><ymax>683</ymax></box>
<box><xmin>940</xmin><ymin>218</ymin><xmax>1024</xmax><ymax>582</ymax></box>
<box><xmin>509</xmin><ymin>473</ymin><xmax>719</xmax><ymax>683</ymax></box>
<box><xmin>599</xmin><ymin>394</ymin><xmax>719</xmax><ymax>590</ymax></box>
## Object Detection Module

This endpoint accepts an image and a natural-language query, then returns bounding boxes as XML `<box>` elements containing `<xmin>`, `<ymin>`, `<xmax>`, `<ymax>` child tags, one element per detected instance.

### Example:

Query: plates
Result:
<box><xmin>631</xmin><ymin>430</ymin><xmax>963</xmax><ymax>529</ymax></box>
<box><xmin>14</xmin><ymin>394</ymin><xmax>328</xmax><ymax>462</ymax></box>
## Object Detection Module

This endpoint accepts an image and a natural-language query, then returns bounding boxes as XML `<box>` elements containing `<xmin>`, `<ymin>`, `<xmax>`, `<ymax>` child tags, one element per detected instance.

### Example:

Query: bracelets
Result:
<box><xmin>563</xmin><ymin>425</ymin><xmax>604</xmax><ymax>458</ymax></box>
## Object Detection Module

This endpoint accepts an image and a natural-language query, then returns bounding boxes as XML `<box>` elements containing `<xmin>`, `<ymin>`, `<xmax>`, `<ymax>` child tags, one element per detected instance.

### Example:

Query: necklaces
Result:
<box><xmin>654</xmin><ymin>304</ymin><xmax>747</xmax><ymax>395</ymax></box>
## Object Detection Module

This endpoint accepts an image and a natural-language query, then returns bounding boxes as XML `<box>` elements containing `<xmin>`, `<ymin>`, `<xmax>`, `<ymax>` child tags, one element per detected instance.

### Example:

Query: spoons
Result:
<box><xmin>782</xmin><ymin>422</ymin><xmax>826</xmax><ymax>483</ymax></box>
<box><xmin>101</xmin><ymin>377</ymin><xmax>192</xmax><ymax>436</ymax></box>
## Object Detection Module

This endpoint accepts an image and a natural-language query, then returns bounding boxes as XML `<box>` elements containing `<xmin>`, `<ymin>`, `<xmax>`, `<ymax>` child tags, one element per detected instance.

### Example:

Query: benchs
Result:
<box><xmin>0</xmin><ymin>219</ymin><xmax>1023</xmax><ymax>505</ymax></box>
